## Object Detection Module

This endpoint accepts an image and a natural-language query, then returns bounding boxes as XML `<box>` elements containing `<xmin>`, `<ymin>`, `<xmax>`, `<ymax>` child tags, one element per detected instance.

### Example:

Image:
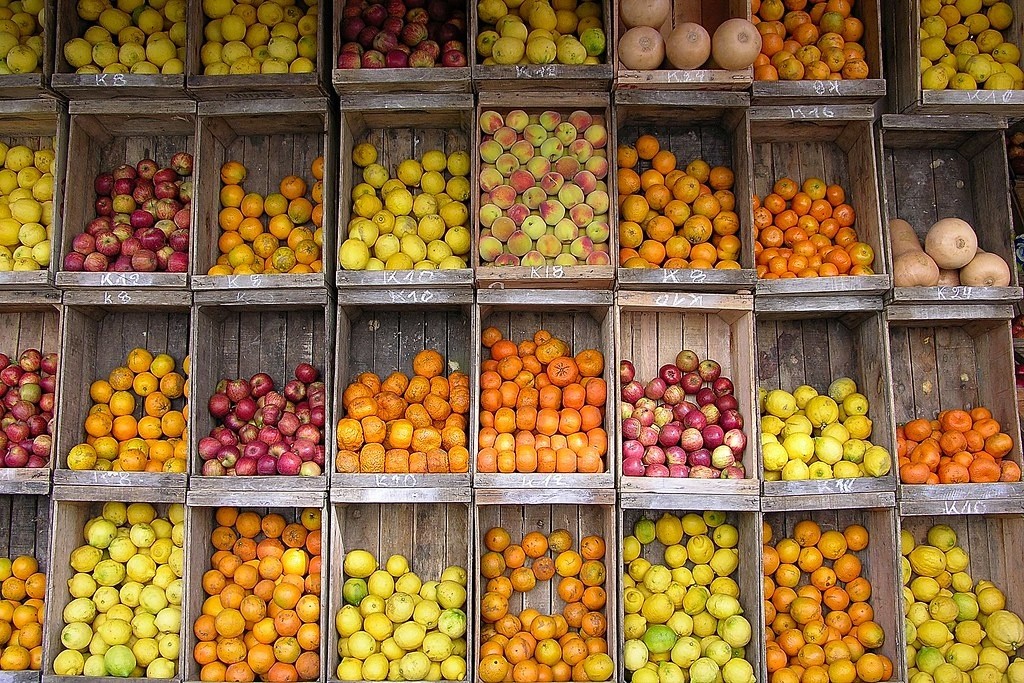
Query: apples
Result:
<box><xmin>199</xmin><ymin>363</ymin><xmax>325</xmax><ymax>477</ymax></box>
<box><xmin>0</xmin><ymin>349</ymin><xmax>57</xmax><ymax>468</ymax></box>
<box><xmin>338</xmin><ymin>0</ymin><xmax>467</xmax><ymax>68</ymax></box>
<box><xmin>63</xmin><ymin>152</ymin><xmax>193</xmax><ymax>272</ymax></box>
<box><xmin>619</xmin><ymin>350</ymin><xmax>746</xmax><ymax>478</ymax></box>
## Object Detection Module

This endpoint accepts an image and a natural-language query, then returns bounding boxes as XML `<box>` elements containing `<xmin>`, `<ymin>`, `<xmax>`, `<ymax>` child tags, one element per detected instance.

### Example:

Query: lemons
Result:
<box><xmin>0</xmin><ymin>0</ymin><xmax>318</xmax><ymax>74</ymax></box>
<box><xmin>339</xmin><ymin>143</ymin><xmax>469</xmax><ymax>271</ymax></box>
<box><xmin>899</xmin><ymin>525</ymin><xmax>1024</xmax><ymax>683</ymax></box>
<box><xmin>622</xmin><ymin>511</ymin><xmax>757</xmax><ymax>683</ymax></box>
<box><xmin>52</xmin><ymin>504</ymin><xmax>184</xmax><ymax>679</ymax></box>
<box><xmin>337</xmin><ymin>550</ymin><xmax>468</xmax><ymax>682</ymax></box>
<box><xmin>759</xmin><ymin>376</ymin><xmax>891</xmax><ymax>480</ymax></box>
<box><xmin>476</xmin><ymin>0</ymin><xmax>607</xmax><ymax>65</ymax></box>
<box><xmin>0</xmin><ymin>135</ymin><xmax>56</xmax><ymax>270</ymax></box>
<box><xmin>920</xmin><ymin>0</ymin><xmax>1024</xmax><ymax>91</ymax></box>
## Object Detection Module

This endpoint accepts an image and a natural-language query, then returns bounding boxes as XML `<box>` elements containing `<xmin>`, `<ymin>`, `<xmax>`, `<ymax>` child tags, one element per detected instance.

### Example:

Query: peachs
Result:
<box><xmin>479</xmin><ymin>109</ymin><xmax>611</xmax><ymax>265</ymax></box>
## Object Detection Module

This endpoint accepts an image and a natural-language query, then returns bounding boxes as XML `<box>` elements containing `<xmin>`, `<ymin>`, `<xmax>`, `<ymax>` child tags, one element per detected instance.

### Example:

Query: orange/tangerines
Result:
<box><xmin>0</xmin><ymin>556</ymin><xmax>47</xmax><ymax>671</ymax></box>
<box><xmin>895</xmin><ymin>406</ymin><xmax>1020</xmax><ymax>484</ymax></box>
<box><xmin>750</xmin><ymin>0</ymin><xmax>870</xmax><ymax>81</ymax></box>
<box><xmin>193</xmin><ymin>506</ymin><xmax>322</xmax><ymax>683</ymax></box>
<box><xmin>753</xmin><ymin>178</ymin><xmax>875</xmax><ymax>278</ymax></box>
<box><xmin>480</xmin><ymin>527</ymin><xmax>614</xmax><ymax>683</ymax></box>
<box><xmin>763</xmin><ymin>522</ymin><xmax>893</xmax><ymax>683</ymax></box>
<box><xmin>336</xmin><ymin>349</ymin><xmax>470</xmax><ymax>473</ymax></box>
<box><xmin>67</xmin><ymin>347</ymin><xmax>189</xmax><ymax>472</ymax></box>
<box><xmin>614</xmin><ymin>134</ymin><xmax>741</xmax><ymax>269</ymax></box>
<box><xmin>478</xmin><ymin>325</ymin><xmax>607</xmax><ymax>473</ymax></box>
<box><xmin>205</xmin><ymin>156</ymin><xmax>323</xmax><ymax>274</ymax></box>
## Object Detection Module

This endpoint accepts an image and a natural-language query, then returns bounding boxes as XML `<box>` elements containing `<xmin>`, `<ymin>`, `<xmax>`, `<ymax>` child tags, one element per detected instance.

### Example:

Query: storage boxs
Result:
<box><xmin>0</xmin><ymin>0</ymin><xmax>1024</xmax><ymax>683</ymax></box>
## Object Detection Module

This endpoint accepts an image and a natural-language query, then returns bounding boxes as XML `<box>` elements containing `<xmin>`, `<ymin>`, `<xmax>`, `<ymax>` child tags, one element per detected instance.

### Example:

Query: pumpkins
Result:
<box><xmin>887</xmin><ymin>216</ymin><xmax>1011</xmax><ymax>287</ymax></box>
